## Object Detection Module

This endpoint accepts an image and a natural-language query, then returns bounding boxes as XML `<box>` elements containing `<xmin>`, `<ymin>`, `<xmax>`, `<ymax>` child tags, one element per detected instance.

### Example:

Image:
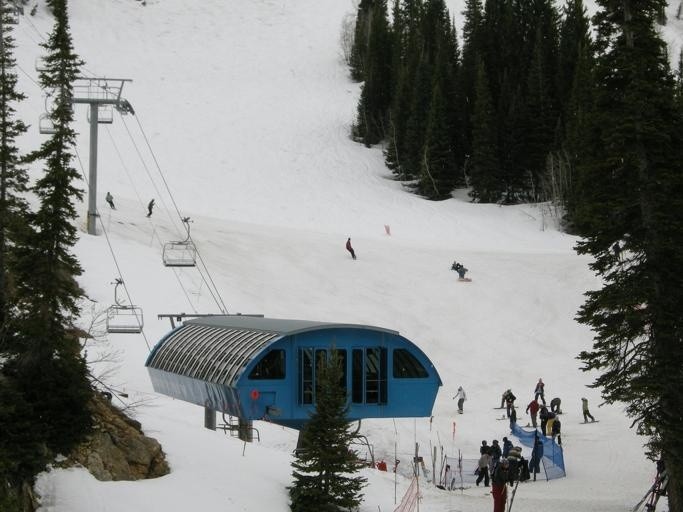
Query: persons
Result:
<box><xmin>533</xmin><ymin>378</ymin><xmax>546</xmax><ymax>406</ymax></box>
<box><xmin>448</xmin><ymin>259</ymin><xmax>468</xmax><ymax>281</ymax></box>
<box><xmin>525</xmin><ymin>399</ymin><xmax>539</xmax><ymax>429</ymax></box>
<box><xmin>104</xmin><ymin>191</ymin><xmax>115</xmax><ymax>210</ymax></box>
<box><xmin>345</xmin><ymin>236</ymin><xmax>356</xmax><ymax>262</ymax></box>
<box><xmin>537</xmin><ymin>403</ymin><xmax>548</xmax><ymax>436</ymax></box>
<box><xmin>509</xmin><ymin>404</ymin><xmax>515</xmax><ymax>436</ymax></box>
<box><xmin>550</xmin><ymin>397</ymin><xmax>561</xmax><ymax>414</ymax></box>
<box><xmin>580</xmin><ymin>397</ymin><xmax>595</xmax><ymax>424</ymax></box>
<box><xmin>452</xmin><ymin>385</ymin><xmax>467</xmax><ymax>416</ymax></box>
<box><xmin>474</xmin><ymin>437</ymin><xmax>523</xmax><ymax>512</ymax></box>
<box><xmin>146</xmin><ymin>197</ymin><xmax>155</xmax><ymax>217</ymax></box>
<box><xmin>550</xmin><ymin>415</ymin><xmax>562</xmax><ymax>446</ymax></box>
<box><xmin>527</xmin><ymin>437</ymin><xmax>542</xmax><ymax>474</ymax></box>
<box><xmin>545</xmin><ymin>412</ymin><xmax>556</xmax><ymax>436</ymax></box>
<box><xmin>500</xmin><ymin>387</ymin><xmax>515</xmax><ymax>419</ymax></box>
